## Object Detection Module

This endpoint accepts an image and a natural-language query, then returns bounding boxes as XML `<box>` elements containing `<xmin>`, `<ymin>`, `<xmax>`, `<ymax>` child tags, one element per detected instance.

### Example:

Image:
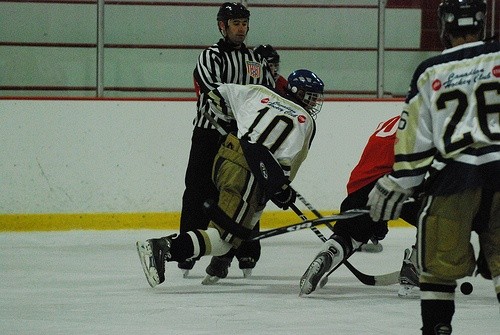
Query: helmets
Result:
<box><xmin>286</xmin><ymin>69</ymin><xmax>324</xmax><ymax>95</ymax></box>
<box><xmin>217</xmin><ymin>2</ymin><xmax>250</xmax><ymax>21</ymax></box>
<box><xmin>253</xmin><ymin>44</ymin><xmax>280</xmax><ymax>64</ymax></box>
<box><xmin>437</xmin><ymin>0</ymin><xmax>486</xmax><ymax>32</ymax></box>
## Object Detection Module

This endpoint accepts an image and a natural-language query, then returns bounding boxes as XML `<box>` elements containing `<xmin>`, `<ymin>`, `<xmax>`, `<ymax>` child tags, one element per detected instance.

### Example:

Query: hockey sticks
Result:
<box><xmin>291</xmin><ymin>185</ymin><xmax>382</xmax><ymax>253</ymax></box>
<box><xmin>289</xmin><ymin>203</ymin><xmax>404</xmax><ymax>286</ymax></box>
<box><xmin>247</xmin><ymin>189</ymin><xmax>424</xmax><ymax>241</ymax></box>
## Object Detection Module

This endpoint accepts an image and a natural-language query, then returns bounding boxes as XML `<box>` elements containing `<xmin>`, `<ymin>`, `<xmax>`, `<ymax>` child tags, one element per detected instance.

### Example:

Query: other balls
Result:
<box><xmin>460</xmin><ymin>282</ymin><xmax>474</xmax><ymax>295</ymax></box>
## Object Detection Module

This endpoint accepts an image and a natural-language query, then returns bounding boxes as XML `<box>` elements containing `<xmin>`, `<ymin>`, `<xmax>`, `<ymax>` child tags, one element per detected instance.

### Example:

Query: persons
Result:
<box><xmin>137</xmin><ymin>2</ymin><xmax>324</xmax><ymax>288</ymax></box>
<box><xmin>303</xmin><ymin>0</ymin><xmax>500</xmax><ymax>335</ymax></box>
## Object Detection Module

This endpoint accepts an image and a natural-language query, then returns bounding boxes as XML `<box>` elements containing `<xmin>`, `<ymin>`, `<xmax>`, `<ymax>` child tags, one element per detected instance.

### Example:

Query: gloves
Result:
<box><xmin>365</xmin><ymin>174</ymin><xmax>413</xmax><ymax>222</ymax></box>
<box><xmin>270</xmin><ymin>185</ymin><xmax>297</xmax><ymax>210</ymax></box>
<box><xmin>217</xmin><ymin>119</ymin><xmax>238</xmax><ymax>149</ymax></box>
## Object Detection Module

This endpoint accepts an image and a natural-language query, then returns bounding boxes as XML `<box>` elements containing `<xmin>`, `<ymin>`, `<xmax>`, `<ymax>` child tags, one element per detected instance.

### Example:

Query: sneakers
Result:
<box><xmin>178</xmin><ymin>256</ymin><xmax>196</xmax><ymax>277</ymax></box>
<box><xmin>136</xmin><ymin>233</ymin><xmax>177</xmax><ymax>287</ymax></box>
<box><xmin>398</xmin><ymin>248</ymin><xmax>420</xmax><ymax>297</ymax></box>
<box><xmin>202</xmin><ymin>256</ymin><xmax>230</xmax><ymax>284</ymax></box>
<box><xmin>238</xmin><ymin>250</ymin><xmax>260</xmax><ymax>278</ymax></box>
<box><xmin>298</xmin><ymin>235</ymin><xmax>349</xmax><ymax>295</ymax></box>
